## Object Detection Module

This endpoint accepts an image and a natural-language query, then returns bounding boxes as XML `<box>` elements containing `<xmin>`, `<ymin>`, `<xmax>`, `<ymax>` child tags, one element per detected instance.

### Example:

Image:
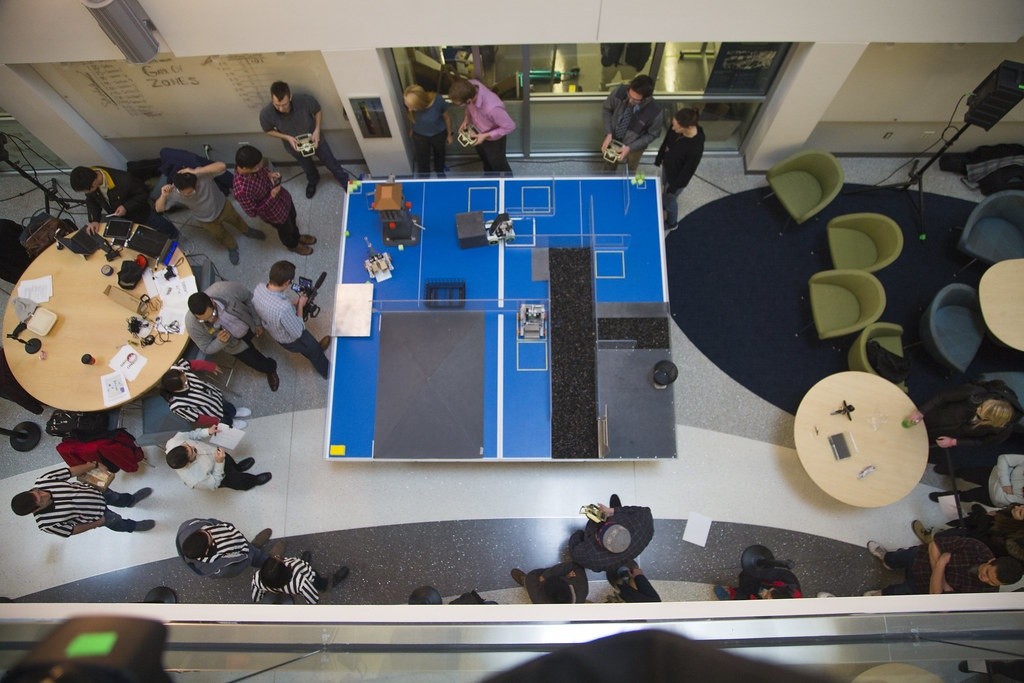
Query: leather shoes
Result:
<box><xmin>238</xmin><ymin>457</ymin><xmax>254</xmax><ymax>471</ymax></box>
<box><xmin>267</xmin><ymin>360</ymin><xmax>279</xmax><ymax>391</ymax></box>
<box><xmin>256</xmin><ymin>472</ymin><xmax>271</xmax><ymax>485</ymax></box>
<box><xmin>317</xmin><ymin>336</ymin><xmax>330</xmax><ymax>351</ymax></box>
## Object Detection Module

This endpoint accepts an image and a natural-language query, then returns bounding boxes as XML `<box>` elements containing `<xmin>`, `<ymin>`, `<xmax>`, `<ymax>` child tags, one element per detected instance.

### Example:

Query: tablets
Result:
<box><xmin>103</xmin><ymin>220</ymin><xmax>133</xmax><ymax>239</ymax></box>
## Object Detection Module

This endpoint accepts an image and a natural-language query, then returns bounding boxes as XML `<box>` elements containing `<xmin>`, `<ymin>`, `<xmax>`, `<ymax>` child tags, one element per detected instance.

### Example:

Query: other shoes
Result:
<box><xmin>132</xmin><ymin>488</ymin><xmax>151</xmax><ymax>503</ymax></box>
<box><xmin>135</xmin><ymin>520</ymin><xmax>155</xmax><ymax>531</ymax></box>
<box><xmin>333</xmin><ymin>567</ymin><xmax>349</xmax><ymax>587</ymax></box>
<box><xmin>610</xmin><ymin>494</ymin><xmax>622</xmax><ymax>508</ymax></box>
<box><xmin>301</xmin><ymin>551</ymin><xmax>311</xmax><ymax>563</ymax></box>
<box><xmin>817</xmin><ymin>591</ymin><xmax>838</xmax><ymax>598</ymax></box>
<box><xmin>511</xmin><ymin>569</ymin><xmax>527</xmax><ymax>586</ymax></box>
<box><xmin>245</xmin><ymin>227</ymin><xmax>265</xmax><ymax>239</ymax></box>
<box><xmin>230</xmin><ymin>245</ymin><xmax>239</xmax><ymax>265</ymax></box>
<box><xmin>269</xmin><ymin>540</ymin><xmax>286</xmax><ymax>558</ymax></box>
<box><xmin>252</xmin><ymin>528</ymin><xmax>272</xmax><ymax>547</ymax></box>
<box><xmin>306</xmin><ymin>177</ymin><xmax>316</xmax><ymax>198</ymax></box>
<box><xmin>664</xmin><ymin>220</ymin><xmax>678</xmax><ymax>232</ymax></box>
<box><xmin>26</xmin><ymin>401</ymin><xmax>44</xmax><ymax>414</ymax></box>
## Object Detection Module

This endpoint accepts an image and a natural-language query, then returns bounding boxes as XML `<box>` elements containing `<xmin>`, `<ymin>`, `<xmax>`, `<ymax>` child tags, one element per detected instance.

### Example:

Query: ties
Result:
<box><xmin>615</xmin><ymin>103</ymin><xmax>634</xmax><ymax>140</ymax></box>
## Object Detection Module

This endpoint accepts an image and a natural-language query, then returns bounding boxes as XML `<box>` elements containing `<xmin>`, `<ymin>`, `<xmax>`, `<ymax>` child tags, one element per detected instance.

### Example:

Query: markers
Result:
<box><xmin>155</xmin><ymin>256</ymin><xmax>160</xmax><ymax>270</ymax></box>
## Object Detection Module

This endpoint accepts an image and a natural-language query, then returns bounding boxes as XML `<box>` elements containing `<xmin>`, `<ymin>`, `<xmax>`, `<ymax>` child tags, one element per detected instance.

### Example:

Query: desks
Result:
<box><xmin>794</xmin><ymin>371</ymin><xmax>930</xmax><ymax>507</ymax></box>
<box><xmin>978</xmin><ymin>258</ymin><xmax>1024</xmax><ymax>353</ymax></box>
<box><xmin>3</xmin><ymin>221</ymin><xmax>196</xmax><ymax>413</ymax></box>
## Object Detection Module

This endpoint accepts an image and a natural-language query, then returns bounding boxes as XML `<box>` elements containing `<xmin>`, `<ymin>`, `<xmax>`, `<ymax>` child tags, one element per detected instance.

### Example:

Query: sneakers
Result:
<box><xmin>234</xmin><ymin>407</ymin><xmax>251</xmax><ymax>417</ymax></box>
<box><xmin>298</xmin><ymin>235</ymin><xmax>316</xmax><ymax>244</ymax></box>
<box><xmin>868</xmin><ymin>540</ymin><xmax>893</xmax><ymax>570</ymax></box>
<box><xmin>289</xmin><ymin>243</ymin><xmax>312</xmax><ymax>255</ymax></box>
<box><xmin>232</xmin><ymin>419</ymin><xmax>247</xmax><ymax>429</ymax></box>
<box><xmin>913</xmin><ymin>520</ymin><xmax>935</xmax><ymax>544</ymax></box>
<box><xmin>863</xmin><ymin>590</ymin><xmax>882</xmax><ymax>596</ymax></box>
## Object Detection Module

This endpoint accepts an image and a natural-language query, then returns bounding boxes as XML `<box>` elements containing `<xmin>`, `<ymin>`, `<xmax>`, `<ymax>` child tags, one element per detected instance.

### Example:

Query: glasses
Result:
<box><xmin>183</xmin><ymin>380</ymin><xmax>188</xmax><ymax>388</ymax></box>
<box><xmin>181</xmin><ymin>441</ymin><xmax>193</xmax><ymax>457</ymax></box>
<box><xmin>198</xmin><ymin>309</ymin><xmax>217</xmax><ymax>323</ymax></box>
<box><xmin>627</xmin><ymin>88</ymin><xmax>642</xmax><ymax>102</ymax></box>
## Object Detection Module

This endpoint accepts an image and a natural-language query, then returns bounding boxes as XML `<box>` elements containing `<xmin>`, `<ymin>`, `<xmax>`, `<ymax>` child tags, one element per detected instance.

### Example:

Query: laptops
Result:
<box><xmin>128</xmin><ymin>225</ymin><xmax>168</xmax><ymax>259</ymax></box>
<box><xmin>53</xmin><ymin>224</ymin><xmax>106</xmax><ymax>255</ymax></box>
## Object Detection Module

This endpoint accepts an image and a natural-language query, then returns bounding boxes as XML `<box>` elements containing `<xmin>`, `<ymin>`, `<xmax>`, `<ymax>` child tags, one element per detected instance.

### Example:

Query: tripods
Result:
<box><xmin>6</xmin><ymin>159</ymin><xmax>87</xmax><ymax>216</ymax></box>
<box><xmin>843</xmin><ymin>122</ymin><xmax>972</xmax><ymax>246</ymax></box>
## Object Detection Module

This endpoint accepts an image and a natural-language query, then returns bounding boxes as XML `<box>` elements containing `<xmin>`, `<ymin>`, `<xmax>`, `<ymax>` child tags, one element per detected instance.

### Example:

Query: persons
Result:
<box><xmin>602</xmin><ymin>75</ymin><xmax>665</xmax><ymax>176</ymax></box>
<box><xmin>569</xmin><ymin>494</ymin><xmax>654</xmax><ymax>572</ymax></box>
<box><xmin>714</xmin><ymin>568</ymin><xmax>801</xmax><ymax>601</ymax></box>
<box><xmin>165</xmin><ymin>425</ymin><xmax>272</xmax><ymax>492</ymax></box>
<box><xmin>185</xmin><ymin>280</ymin><xmax>278</xmax><ymax>391</ymax></box>
<box><xmin>655</xmin><ymin>109</ymin><xmax>705</xmax><ymax>229</ymax></box>
<box><xmin>70</xmin><ymin>166</ymin><xmax>194</xmax><ymax>254</ymax></box>
<box><xmin>251</xmin><ymin>261</ymin><xmax>330</xmax><ymax>379</ymax></box>
<box><xmin>511</xmin><ymin>562</ymin><xmax>589</xmax><ymax>603</ymax></box>
<box><xmin>260</xmin><ymin>82</ymin><xmax>347</xmax><ymax>198</ymax></box>
<box><xmin>176</xmin><ymin>519</ymin><xmax>348</xmax><ymax>604</ymax></box>
<box><xmin>160</xmin><ymin>360</ymin><xmax>251</xmax><ymax>429</ymax></box>
<box><xmin>154</xmin><ymin>162</ymin><xmax>265</xmax><ymax>265</ymax></box>
<box><xmin>449</xmin><ymin>78</ymin><xmax>515</xmax><ymax>176</ymax></box>
<box><xmin>606</xmin><ymin>568</ymin><xmax>661</xmax><ymax>603</ymax></box>
<box><xmin>816</xmin><ymin>381</ymin><xmax>1024</xmax><ymax>598</ymax></box>
<box><xmin>404</xmin><ymin>85</ymin><xmax>453</xmax><ymax>178</ymax></box>
<box><xmin>12</xmin><ymin>462</ymin><xmax>155</xmax><ymax>537</ymax></box>
<box><xmin>233</xmin><ymin>146</ymin><xmax>317</xmax><ymax>255</ymax></box>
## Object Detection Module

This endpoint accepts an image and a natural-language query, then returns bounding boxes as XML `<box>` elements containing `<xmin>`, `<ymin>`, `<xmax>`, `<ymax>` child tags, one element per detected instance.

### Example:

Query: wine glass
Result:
<box><xmin>273</xmin><ymin>168</ymin><xmax>279</xmax><ymax>189</ymax></box>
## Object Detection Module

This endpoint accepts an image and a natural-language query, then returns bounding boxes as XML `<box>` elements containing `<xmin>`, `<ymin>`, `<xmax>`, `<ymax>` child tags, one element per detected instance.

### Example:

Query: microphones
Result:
<box><xmin>307</xmin><ymin>271</ymin><xmax>327</xmax><ymax>305</ymax></box>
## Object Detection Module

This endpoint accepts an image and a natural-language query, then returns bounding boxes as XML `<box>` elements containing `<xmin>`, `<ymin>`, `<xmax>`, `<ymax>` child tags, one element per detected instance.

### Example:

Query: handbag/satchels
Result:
<box><xmin>865</xmin><ymin>340</ymin><xmax>912</xmax><ymax>384</ymax></box>
<box><xmin>19</xmin><ymin>213</ymin><xmax>60</xmax><ymax>256</ymax></box>
<box><xmin>46</xmin><ymin>409</ymin><xmax>109</xmax><ymax>442</ymax></box>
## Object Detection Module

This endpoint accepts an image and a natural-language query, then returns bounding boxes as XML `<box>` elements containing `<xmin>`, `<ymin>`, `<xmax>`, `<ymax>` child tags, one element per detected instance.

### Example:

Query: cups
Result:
<box><xmin>901</xmin><ymin>414</ymin><xmax>917</xmax><ymax>428</ymax></box>
<box><xmin>101</xmin><ymin>265</ymin><xmax>113</xmax><ymax>276</ymax></box>
<box><xmin>81</xmin><ymin>354</ymin><xmax>96</xmax><ymax>365</ymax></box>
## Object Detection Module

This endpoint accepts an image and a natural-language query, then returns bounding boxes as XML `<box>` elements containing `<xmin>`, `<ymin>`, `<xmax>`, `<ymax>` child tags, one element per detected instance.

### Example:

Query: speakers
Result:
<box><xmin>0</xmin><ymin>132</ymin><xmax>9</xmax><ymax>161</ymax></box>
<box><xmin>964</xmin><ymin>60</ymin><xmax>1024</xmax><ymax>132</ymax></box>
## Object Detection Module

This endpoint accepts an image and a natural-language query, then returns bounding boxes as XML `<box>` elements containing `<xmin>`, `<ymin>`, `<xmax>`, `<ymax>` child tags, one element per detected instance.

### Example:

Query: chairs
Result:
<box><xmin>795</xmin><ymin>268</ymin><xmax>886</xmax><ymax>341</ymax></box>
<box><xmin>949</xmin><ymin>188</ymin><xmax>1024</xmax><ymax>280</ymax></box>
<box><xmin>810</xmin><ymin>211</ymin><xmax>904</xmax><ymax>276</ymax></box>
<box><xmin>185</xmin><ymin>253</ymin><xmax>240</xmax><ymax>397</ymax></box>
<box><xmin>846</xmin><ymin>320</ymin><xmax>912</xmax><ymax>391</ymax></box>
<box><xmin>916</xmin><ymin>283</ymin><xmax>985</xmax><ymax>379</ymax></box>
<box><xmin>761</xmin><ymin>148</ymin><xmax>844</xmax><ymax>233</ymax></box>
